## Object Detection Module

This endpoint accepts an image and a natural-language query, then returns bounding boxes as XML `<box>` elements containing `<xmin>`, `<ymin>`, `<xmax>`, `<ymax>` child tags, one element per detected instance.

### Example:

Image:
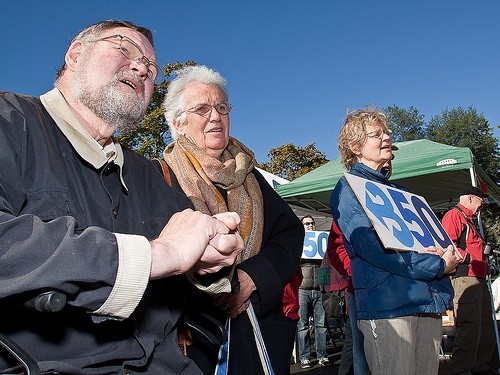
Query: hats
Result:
<box><xmin>458</xmin><ymin>185</ymin><xmax>489</xmax><ymax>198</ymax></box>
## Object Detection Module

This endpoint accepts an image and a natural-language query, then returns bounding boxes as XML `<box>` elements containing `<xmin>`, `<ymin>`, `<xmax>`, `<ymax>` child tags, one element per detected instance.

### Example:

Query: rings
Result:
<box><xmin>211</xmin><ymin>217</ymin><xmax>217</xmax><ymax>219</ymax></box>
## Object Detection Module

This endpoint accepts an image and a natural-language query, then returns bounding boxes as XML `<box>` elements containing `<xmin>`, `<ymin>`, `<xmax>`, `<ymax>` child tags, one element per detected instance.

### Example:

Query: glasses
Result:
<box><xmin>304</xmin><ymin>222</ymin><xmax>315</xmax><ymax>226</ymax></box>
<box><xmin>177</xmin><ymin>102</ymin><xmax>233</xmax><ymax>116</ymax></box>
<box><xmin>366</xmin><ymin>129</ymin><xmax>392</xmax><ymax>138</ymax></box>
<box><xmin>391</xmin><ymin>153</ymin><xmax>395</xmax><ymax>160</ymax></box>
<box><xmin>88</xmin><ymin>34</ymin><xmax>165</xmax><ymax>86</ymax></box>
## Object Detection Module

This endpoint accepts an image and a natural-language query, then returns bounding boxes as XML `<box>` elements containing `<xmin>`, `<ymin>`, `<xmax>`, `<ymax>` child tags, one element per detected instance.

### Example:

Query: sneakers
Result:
<box><xmin>300</xmin><ymin>360</ymin><xmax>312</xmax><ymax>368</ymax></box>
<box><xmin>317</xmin><ymin>357</ymin><xmax>331</xmax><ymax>367</ymax></box>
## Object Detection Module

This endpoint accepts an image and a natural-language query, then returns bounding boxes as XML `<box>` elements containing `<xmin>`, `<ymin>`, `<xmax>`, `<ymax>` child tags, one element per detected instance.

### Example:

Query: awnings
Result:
<box><xmin>274</xmin><ymin>140</ymin><xmax>500</xmax><ymax>358</ymax></box>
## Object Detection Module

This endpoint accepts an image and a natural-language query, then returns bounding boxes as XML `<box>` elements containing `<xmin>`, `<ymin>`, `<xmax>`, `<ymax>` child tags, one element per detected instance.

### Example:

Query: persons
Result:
<box><xmin>298</xmin><ymin>215</ymin><xmax>332</xmax><ymax>367</ymax></box>
<box><xmin>440</xmin><ymin>186</ymin><xmax>500</xmax><ymax>375</ymax></box>
<box><xmin>0</xmin><ymin>20</ymin><xmax>245</xmax><ymax>375</ymax></box>
<box><xmin>151</xmin><ymin>68</ymin><xmax>305</xmax><ymax>375</ymax></box>
<box><xmin>328</xmin><ymin>146</ymin><xmax>398</xmax><ymax>375</ymax></box>
<box><xmin>330</xmin><ymin>108</ymin><xmax>459</xmax><ymax>375</ymax></box>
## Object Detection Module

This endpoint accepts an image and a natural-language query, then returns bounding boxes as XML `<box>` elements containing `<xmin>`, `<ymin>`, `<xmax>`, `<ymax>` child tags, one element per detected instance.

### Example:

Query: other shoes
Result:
<box><xmin>479</xmin><ymin>369</ymin><xmax>500</xmax><ymax>375</ymax></box>
